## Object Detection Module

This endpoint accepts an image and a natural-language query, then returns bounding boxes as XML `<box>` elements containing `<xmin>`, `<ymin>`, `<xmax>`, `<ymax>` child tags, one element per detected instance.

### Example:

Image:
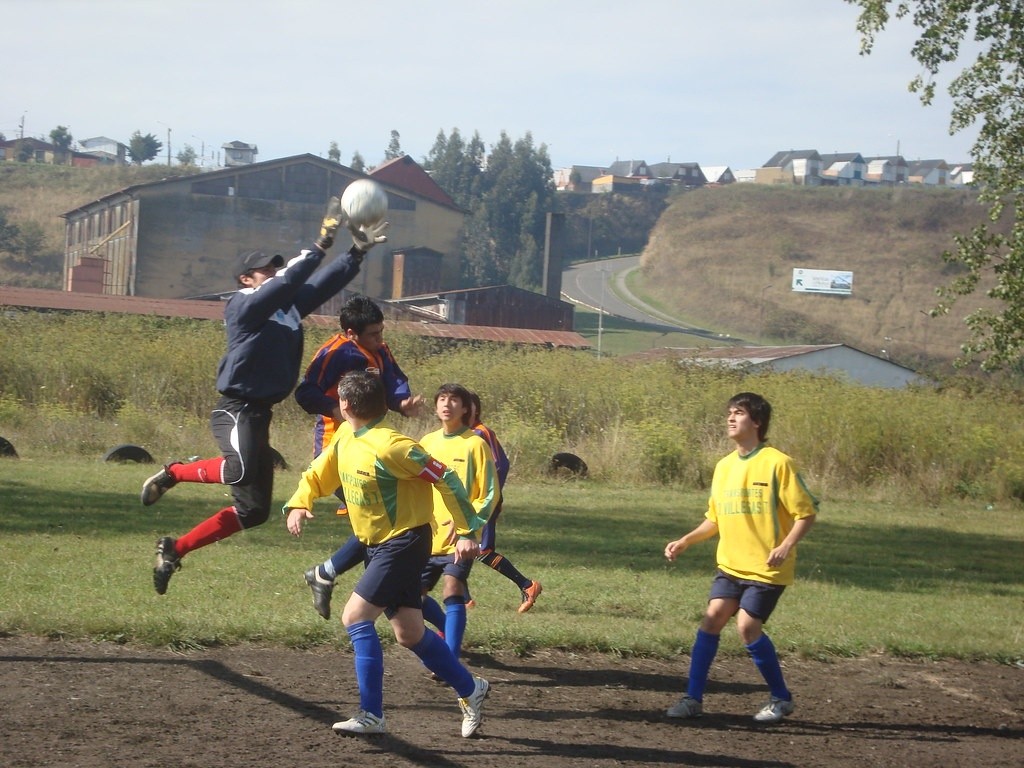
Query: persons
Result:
<box><xmin>665</xmin><ymin>391</ymin><xmax>820</xmax><ymax>721</ymax></box>
<box><xmin>283</xmin><ymin>369</ymin><xmax>493</xmax><ymax>739</ymax></box>
<box><xmin>140</xmin><ymin>196</ymin><xmax>386</xmax><ymax>595</ymax></box>
<box><xmin>419</xmin><ymin>383</ymin><xmax>505</xmax><ymax>686</ymax></box>
<box><xmin>294</xmin><ymin>295</ymin><xmax>424</xmax><ymax>470</ymax></box>
<box><xmin>465</xmin><ymin>389</ymin><xmax>543</xmax><ymax>614</ymax></box>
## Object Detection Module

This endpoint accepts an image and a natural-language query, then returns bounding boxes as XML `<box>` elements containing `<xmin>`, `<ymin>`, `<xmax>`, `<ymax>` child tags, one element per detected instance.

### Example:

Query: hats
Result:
<box><xmin>232</xmin><ymin>250</ymin><xmax>284</xmax><ymax>278</ymax></box>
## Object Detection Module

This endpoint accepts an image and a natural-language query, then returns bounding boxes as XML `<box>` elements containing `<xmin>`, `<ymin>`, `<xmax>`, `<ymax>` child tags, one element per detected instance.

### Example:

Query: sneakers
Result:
<box><xmin>141</xmin><ymin>461</ymin><xmax>184</xmax><ymax>506</ymax></box>
<box><xmin>303</xmin><ymin>566</ymin><xmax>336</xmax><ymax>621</ymax></box>
<box><xmin>458</xmin><ymin>677</ymin><xmax>491</xmax><ymax>737</ymax></box>
<box><xmin>667</xmin><ymin>698</ymin><xmax>703</xmax><ymax>717</ymax></box>
<box><xmin>518</xmin><ymin>580</ymin><xmax>542</xmax><ymax>613</ymax></box>
<box><xmin>332</xmin><ymin>710</ymin><xmax>386</xmax><ymax>734</ymax></box>
<box><xmin>465</xmin><ymin>600</ymin><xmax>475</xmax><ymax>609</ymax></box>
<box><xmin>753</xmin><ymin>696</ymin><xmax>795</xmax><ymax>721</ymax></box>
<box><xmin>153</xmin><ymin>537</ymin><xmax>182</xmax><ymax>594</ymax></box>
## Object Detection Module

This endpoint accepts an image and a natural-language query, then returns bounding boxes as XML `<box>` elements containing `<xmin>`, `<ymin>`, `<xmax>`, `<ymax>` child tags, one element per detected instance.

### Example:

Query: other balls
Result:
<box><xmin>341</xmin><ymin>180</ymin><xmax>387</xmax><ymax>225</ymax></box>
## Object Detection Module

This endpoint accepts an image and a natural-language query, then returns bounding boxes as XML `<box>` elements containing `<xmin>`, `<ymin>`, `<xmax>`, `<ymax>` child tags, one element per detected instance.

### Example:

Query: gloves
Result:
<box><xmin>317</xmin><ymin>197</ymin><xmax>342</xmax><ymax>247</ymax></box>
<box><xmin>349</xmin><ymin>217</ymin><xmax>389</xmax><ymax>256</ymax></box>
